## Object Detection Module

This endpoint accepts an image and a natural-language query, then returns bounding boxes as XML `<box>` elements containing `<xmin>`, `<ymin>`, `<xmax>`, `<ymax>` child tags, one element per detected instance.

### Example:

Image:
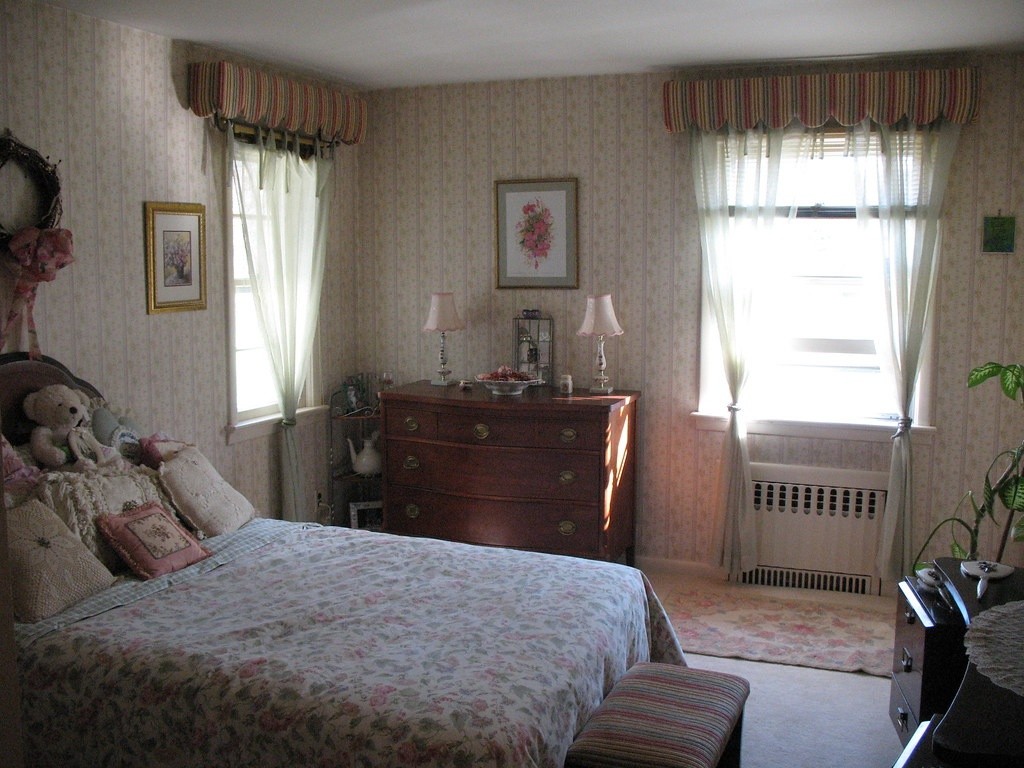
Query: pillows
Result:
<box><xmin>1</xmin><ymin>497</ymin><xmax>119</xmax><ymax>623</ymax></box>
<box><xmin>95</xmin><ymin>498</ymin><xmax>214</xmax><ymax>580</ymax></box>
<box><xmin>1</xmin><ymin>395</ymin><xmax>186</xmax><ymax>577</ymax></box>
<box><xmin>156</xmin><ymin>445</ymin><xmax>255</xmax><ymax>537</ymax></box>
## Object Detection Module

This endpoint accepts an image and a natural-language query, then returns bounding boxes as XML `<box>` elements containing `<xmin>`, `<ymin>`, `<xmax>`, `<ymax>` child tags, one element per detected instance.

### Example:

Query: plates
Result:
<box><xmin>473</xmin><ymin>375</ymin><xmax>543</xmax><ymax>396</ymax></box>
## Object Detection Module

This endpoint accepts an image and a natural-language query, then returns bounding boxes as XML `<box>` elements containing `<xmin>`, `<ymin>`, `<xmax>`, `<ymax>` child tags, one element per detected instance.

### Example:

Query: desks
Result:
<box><xmin>932</xmin><ymin>556</ymin><xmax>1024</xmax><ymax>625</ymax></box>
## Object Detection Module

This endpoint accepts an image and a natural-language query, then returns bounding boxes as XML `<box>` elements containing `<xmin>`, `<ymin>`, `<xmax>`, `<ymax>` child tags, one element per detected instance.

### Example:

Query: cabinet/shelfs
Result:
<box><xmin>888</xmin><ymin>573</ymin><xmax>967</xmax><ymax>750</ymax></box>
<box><xmin>328</xmin><ymin>387</ymin><xmax>384</xmax><ymax>533</ymax></box>
<box><xmin>377</xmin><ymin>379</ymin><xmax>641</xmax><ymax>567</ymax></box>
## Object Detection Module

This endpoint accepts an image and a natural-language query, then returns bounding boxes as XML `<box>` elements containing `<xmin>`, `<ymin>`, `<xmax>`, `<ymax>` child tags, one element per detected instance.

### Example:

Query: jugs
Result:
<box><xmin>347</xmin><ymin>431</ymin><xmax>382</xmax><ymax>474</ymax></box>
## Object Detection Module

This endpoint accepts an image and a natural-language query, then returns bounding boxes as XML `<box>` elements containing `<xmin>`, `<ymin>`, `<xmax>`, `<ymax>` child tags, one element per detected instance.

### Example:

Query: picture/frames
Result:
<box><xmin>143</xmin><ymin>200</ymin><xmax>208</xmax><ymax>315</ymax></box>
<box><xmin>491</xmin><ymin>179</ymin><xmax>580</xmax><ymax>290</ymax></box>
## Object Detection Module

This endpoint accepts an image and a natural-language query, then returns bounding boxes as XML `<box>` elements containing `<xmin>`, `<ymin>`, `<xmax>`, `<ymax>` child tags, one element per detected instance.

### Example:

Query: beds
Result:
<box><xmin>1</xmin><ymin>347</ymin><xmax>689</xmax><ymax>768</ymax></box>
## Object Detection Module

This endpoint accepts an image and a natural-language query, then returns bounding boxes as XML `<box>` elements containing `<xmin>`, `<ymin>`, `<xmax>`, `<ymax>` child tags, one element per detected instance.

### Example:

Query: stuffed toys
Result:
<box><xmin>25</xmin><ymin>384</ymin><xmax>123</xmax><ymax>471</ymax></box>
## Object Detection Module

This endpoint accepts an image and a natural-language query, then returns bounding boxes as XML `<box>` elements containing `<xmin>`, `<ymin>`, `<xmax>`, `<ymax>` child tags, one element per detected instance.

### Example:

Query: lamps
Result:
<box><xmin>577</xmin><ymin>295</ymin><xmax>625</xmax><ymax>394</ymax></box>
<box><xmin>423</xmin><ymin>291</ymin><xmax>463</xmax><ymax>386</ymax></box>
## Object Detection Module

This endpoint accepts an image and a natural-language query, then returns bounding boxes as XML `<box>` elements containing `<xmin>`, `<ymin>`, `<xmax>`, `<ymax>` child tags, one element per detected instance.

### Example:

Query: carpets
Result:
<box><xmin>638</xmin><ymin>571</ymin><xmax>898</xmax><ymax>677</ymax></box>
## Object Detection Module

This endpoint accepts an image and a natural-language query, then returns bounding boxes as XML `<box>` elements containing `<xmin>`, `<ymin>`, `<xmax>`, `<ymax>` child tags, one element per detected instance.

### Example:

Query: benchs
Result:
<box><xmin>570</xmin><ymin>662</ymin><xmax>749</xmax><ymax>767</ymax></box>
